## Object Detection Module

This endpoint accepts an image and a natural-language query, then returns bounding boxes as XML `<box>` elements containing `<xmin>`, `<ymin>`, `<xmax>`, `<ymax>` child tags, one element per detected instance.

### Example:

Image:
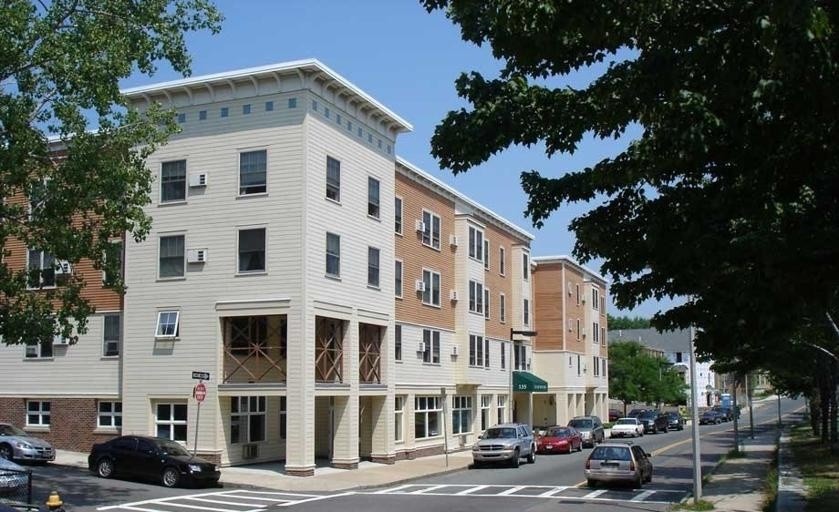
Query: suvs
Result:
<box><xmin>472</xmin><ymin>422</ymin><xmax>537</xmax><ymax>468</ymax></box>
<box><xmin>568</xmin><ymin>415</ymin><xmax>605</xmax><ymax>448</ymax></box>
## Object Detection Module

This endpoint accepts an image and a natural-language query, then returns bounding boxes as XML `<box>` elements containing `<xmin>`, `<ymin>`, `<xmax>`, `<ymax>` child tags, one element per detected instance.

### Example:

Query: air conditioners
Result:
<box><xmin>53</xmin><ymin>257</ymin><xmax>72</xmax><ymax>276</ymax></box>
<box><xmin>417</xmin><ymin>341</ymin><xmax>461</xmax><ymax>357</ymax></box>
<box><xmin>449</xmin><ymin>288</ymin><xmax>458</xmax><ymax>300</ymax></box>
<box><xmin>187</xmin><ymin>249</ymin><xmax>207</xmax><ymax>264</ymax></box>
<box><xmin>51</xmin><ymin>333</ymin><xmax>71</xmax><ymax>345</ymax></box>
<box><xmin>415</xmin><ymin>280</ymin><xmax>426</xmax><ymax>292</ymax></box>
<box><xmin>450</xmin><ymin>235</ymin><xmax>457</xmax><ymax>246</ymax></box>
<box><xmin>244</xmin><ymin>445</ymin><xmax>258</xmax><ymax>458</ymax></box>
<box><xmin>189</xmin><ymin>173</ymin><xmax>208</xmax><ymax>187</ymax></box>
<box><xmin>415</xmin><ymin>219</ymin><xmax>427</xmax><ymax>233</ymax></box>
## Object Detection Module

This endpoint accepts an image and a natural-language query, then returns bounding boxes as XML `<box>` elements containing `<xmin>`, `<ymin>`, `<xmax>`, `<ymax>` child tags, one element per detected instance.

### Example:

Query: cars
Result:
<box><xmin>583</xmin><ymin>440</ymin><xmax>654</xmax><ymax>488</ymax></box>
<box><xmin>609</xmin><ymin>417</ymin><xmax>645</xmax><ymax>438</ymax></box>
<box><xmin>0</xmin><ymin>422</ymin><xmax>56</xmax><ymax>463</ymax></box>
<box><xmin>628</xmin><ymin>406</ymin><xmax>741</xmax><ymax>434</ymax></box>
<box><xmin>87</xmin><ymin>435</ymin><xmax>221</xmax><ymax>488</ymax></box>
<box><xmin>538</xmin><ymin>426</ymin><xmax>583</xmax><ymax>454</ymax></box>
